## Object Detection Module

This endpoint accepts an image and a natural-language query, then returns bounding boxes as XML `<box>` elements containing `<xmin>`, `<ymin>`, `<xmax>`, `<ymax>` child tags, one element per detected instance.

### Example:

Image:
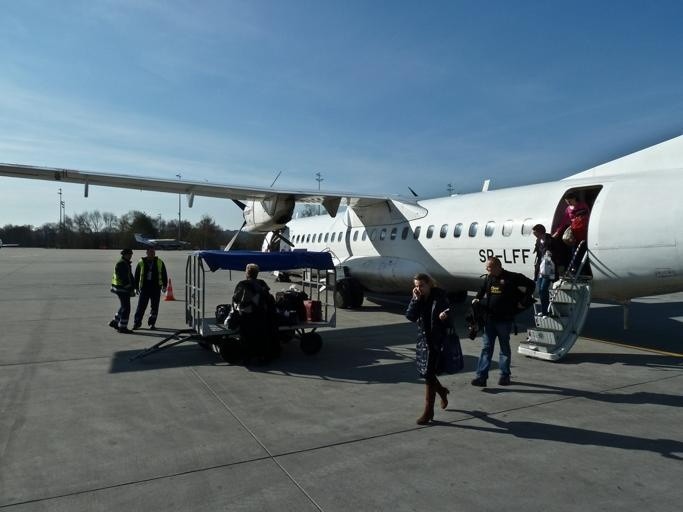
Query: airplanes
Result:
<box><xmin>134</xmin><ymin>232</ymin><xmax>193</xmax><ymax>250</ymax></box>
<box><xmin>0</xmin><ymin>239</ymin><xmax>20</xmax><ymax>247</ymax></box>
<box><xmin>0</xmin><ymin>124</ymin><xmax>683</xmax><ymax>365</ymax></box>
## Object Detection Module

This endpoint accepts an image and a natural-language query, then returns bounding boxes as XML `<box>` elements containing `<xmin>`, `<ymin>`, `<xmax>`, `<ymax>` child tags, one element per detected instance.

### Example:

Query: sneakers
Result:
<box><xmin>537</xmin><ymin>312</ymin><xmax>548</xmax><ymax>319</ymax></box>
<box><xmin>471</xmin><ymin>373</ymin><xmax>511</xmax><ymax>387</ymax></box>
<box><xmin>108</xmin><ymin>320</ymin><xmax>156</xmax><ymax>334</ymax></box>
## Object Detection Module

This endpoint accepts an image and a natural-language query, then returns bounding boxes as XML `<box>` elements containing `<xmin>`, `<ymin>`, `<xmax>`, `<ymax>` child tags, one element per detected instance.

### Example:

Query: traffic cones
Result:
<box><xmin>162</xmin><ymin>276</ymin><xmax>175</xmax><ymax>301</ymax></box>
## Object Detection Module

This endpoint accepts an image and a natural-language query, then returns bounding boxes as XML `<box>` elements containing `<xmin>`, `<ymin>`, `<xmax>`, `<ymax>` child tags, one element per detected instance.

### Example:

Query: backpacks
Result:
<box><xmin>465</xmin><ymin>300</ymin><xmax>489</xmax><ymax>339</ymax></box>
<box><xmin>562</xmin><ymin>225</ymin><xmax>576</xmax><ymax>248</ymax></box>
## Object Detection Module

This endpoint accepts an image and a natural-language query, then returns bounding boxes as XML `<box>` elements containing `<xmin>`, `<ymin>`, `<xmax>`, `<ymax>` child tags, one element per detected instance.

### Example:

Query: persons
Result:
<box><xmin>551</xmin><ymin>193</ymin><xmax>590</xmax><ymax>275</ymax></box>
<box><xmin>532</xmin><ymin>224</ymin><xmax>559</xmax><ymax>319</ymax></box>
<box><xmin>133</xmin><ymin>246</ymin><xmax>168</xmax><ymax>330</ymax></box>
<box><xmin>108</xmin><ymin>248</ymin><xmax>135</xmax><ymax>334</ymax></box>
<box><xmin>405</xmin><ymin>273</ymin><xmax>457</xmax><ymax>425</ymax></box>
<box><xmin>232</xmin><ymin>263</ymin><xmax>270</xmax><ymax>366</ymax></box>
<box><xmin>471</xmin><ymin>257</ymin><xmax>537</xmax><ymax>386</ymax></box>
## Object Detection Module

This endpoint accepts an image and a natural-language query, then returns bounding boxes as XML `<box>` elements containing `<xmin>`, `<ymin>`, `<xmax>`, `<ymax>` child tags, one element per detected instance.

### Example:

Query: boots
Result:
<box><xmin>435</xmin><ymin>380</ymin><xmax>450</xmax><ymax>410</ymax></box>
<box><xmin>416</xmin><ymin>384</ymin><xmax>437</xmax><ymax>426</ymax></box>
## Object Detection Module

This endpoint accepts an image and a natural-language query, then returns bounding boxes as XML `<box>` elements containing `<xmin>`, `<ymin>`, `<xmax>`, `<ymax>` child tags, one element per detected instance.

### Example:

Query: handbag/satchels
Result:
<box><xmin>216</xmin><ymin>304</ymin><xmax>232</xmax><ymax>323</ymax></box>
<box><xmin>539</xmin><ymin>252</ymin><xmax>555</xmax><ymax>281</ymax></box>
<box><xmin>224</xmin><ymin>309</ymin><xmax>241</xmax><ymax>330</ymax></box>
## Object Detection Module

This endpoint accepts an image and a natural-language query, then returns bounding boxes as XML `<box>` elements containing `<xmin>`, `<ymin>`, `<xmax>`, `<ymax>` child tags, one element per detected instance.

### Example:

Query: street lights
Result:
<box><xmin>57</xmin><ymin>183</ymin><xmax>62</xmax><ymax>248</ymax></box>
<box><xmin>61</xmin><ymin>198</ymin><xmax>66</xmax><ymax>247</ymax></box>
<box><xmin>174</xmin><ymin>171</ymin><xmax>183</xmax><ymax>252</ymax></box>
<box><xmin>444</xmin><ymin>180</ymin><xmax>453</xmax><ymax>195</ymax></box>
<box><xmin>313</xmin><ymin>169</ymin><xmax>323</xmax><ymax>190</ymax></box>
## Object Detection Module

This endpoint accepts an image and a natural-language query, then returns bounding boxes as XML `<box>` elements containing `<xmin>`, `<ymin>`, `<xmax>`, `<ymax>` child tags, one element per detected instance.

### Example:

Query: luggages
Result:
<box><xmin>275</xmin><ymin>290</ymin><xmax>322</xmax><ymax>326</ymax></box>
